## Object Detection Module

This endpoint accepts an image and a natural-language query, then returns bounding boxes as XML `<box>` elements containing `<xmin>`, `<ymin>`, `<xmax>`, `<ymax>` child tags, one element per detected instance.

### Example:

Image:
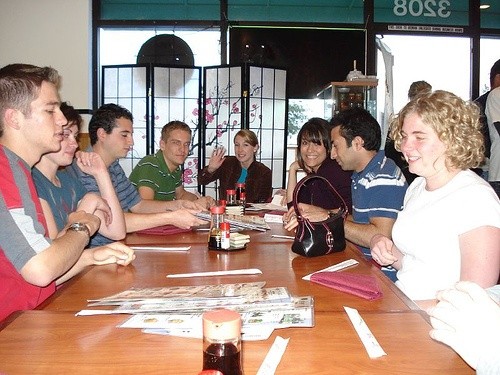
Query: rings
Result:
<box><xmin>375</xmin><ymin>258</ymin><xmax>379</xmax><ymax>262</ymax></box>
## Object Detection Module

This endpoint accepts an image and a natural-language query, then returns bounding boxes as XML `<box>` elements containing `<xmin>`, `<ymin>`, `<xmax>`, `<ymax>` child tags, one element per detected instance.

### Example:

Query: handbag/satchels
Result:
<box><xmin>291</xmin><ymin>174</ymin><xmax>348</xmax><ymax>257</ymax></box>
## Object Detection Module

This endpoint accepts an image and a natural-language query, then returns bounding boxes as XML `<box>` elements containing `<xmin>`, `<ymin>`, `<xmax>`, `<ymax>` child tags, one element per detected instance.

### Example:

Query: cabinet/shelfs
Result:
<box><xmin>323</xmin><ymin>81</ymin><xmax>378</xmax><ymax>123</ymax></box>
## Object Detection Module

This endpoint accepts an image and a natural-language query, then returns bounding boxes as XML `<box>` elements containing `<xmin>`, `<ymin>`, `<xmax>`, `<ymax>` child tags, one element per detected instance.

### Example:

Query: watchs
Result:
<box><xmin>67</xmin><ymin>222</ymin><xmax>91</xmax><ymax>246</ymax></box>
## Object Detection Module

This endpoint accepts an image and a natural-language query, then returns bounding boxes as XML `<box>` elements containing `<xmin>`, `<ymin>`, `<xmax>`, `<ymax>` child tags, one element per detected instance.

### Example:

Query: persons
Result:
<box><xmin>72</xmin><ymin>103</ymin><xmax>212</xmax><ymax>248</ymax></box>
<box><xmin>371</xmin><ymin>89</ymin><xmax>500</xmax><ymax>316</ymax></box>
<box><xmin>128</xmin><ymin>119</ymin><xmax>216</xmax><ymax>212</ymax></box>
<box><xmin>277</xmin><ymin>117</ymin><xmax>353</xmax><ymax>215</ymax></box>
<box><xmin>31</xmin><ymin>99</ymin><xmax>127</xmax><ymax>245</ymax></box>
<box><xmin>429</xmin><ymin>280</ymin><xmax>500</xmax><ymax>375</ymax></box>
<box><xmin>472</xmin><ymin>58</ymin><xmax>500</xmax><ymax>197</ymax></box>
<box><xmin>198</xmin><ymin>130</ymin><xmax>273</xmax><ymax>203</ymax></box>
<box><xmin>282</xmin><ymin>108</ymin><xmax>410</xmax><ymax>284</ymax></box>
<box><xmin>385</xmin><ymin>80</ymin><xmax>432</xmax><ymax>184</ymax></box>
<box><xmin>0</xmin><ymin>63</ymin><xmax>137</xmax><ymax>323</ymax></box>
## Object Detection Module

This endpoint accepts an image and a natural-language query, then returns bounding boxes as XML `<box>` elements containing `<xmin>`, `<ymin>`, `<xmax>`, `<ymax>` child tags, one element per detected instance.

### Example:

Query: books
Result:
<box><xmin>189</xmin><ymin>201</ymin><xmax>270</xmax><ymax>234</ymax></box>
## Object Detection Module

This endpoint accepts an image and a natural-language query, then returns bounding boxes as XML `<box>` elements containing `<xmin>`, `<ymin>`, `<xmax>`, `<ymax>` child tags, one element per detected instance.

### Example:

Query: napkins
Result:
<box><xmin>308</xmin><ymin>268</ymin><xmax>380</xmax><ymax>302</ymax></box>
<box><xmin>136</xmin><ymin>224</ymin><xmax>197</xmax><ymax>238</ymax></box>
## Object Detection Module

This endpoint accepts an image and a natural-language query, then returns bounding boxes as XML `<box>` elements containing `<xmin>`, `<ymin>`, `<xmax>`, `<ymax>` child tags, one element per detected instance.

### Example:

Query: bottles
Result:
<box><xmin>219</xmin><ymin>222</ymin><xmax>231</xmax><ymax>250</ymax></box>
<box><xmin>207</xmin><ymin>206</ymin><xmax>225</xmax><ymax>250</ymax></box>
<box><xmin>202</xmin><ymin>309</ymin><xmax>244</xmax><ymax>375</ymax></box>
<box><xmin>220</xmin><ymin>184</ymin><xmax>247</xmax><ymax>208</ymax></box>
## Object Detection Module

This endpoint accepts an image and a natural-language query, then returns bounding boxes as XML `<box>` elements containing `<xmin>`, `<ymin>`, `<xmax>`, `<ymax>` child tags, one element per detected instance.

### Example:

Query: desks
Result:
<box><xmin>0</xmin><ymin>203</ymin><xmax>477</xmax><ymax>374</ymax></box>
